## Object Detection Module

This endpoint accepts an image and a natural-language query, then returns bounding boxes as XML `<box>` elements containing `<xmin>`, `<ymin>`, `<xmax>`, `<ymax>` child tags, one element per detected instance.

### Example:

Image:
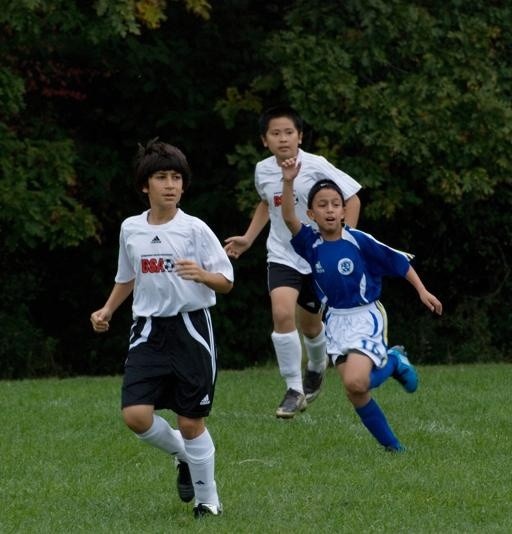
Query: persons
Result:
<box><xmin>282</xmin><ymin>156</ymin><xmax>443</xmax><ymax>451</ymax></box>
<box><xmin>222</xmin><ymin>110</ymin><xmax>362</xmax><ymax>420</ymax></box>
<box><xmin>91</xmin><ymin>141</ymin><xmax>234</xmax><ymax>520</ymax></box>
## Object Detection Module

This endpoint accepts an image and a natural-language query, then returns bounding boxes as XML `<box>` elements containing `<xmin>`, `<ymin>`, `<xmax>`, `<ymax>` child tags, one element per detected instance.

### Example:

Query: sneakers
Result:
<box><xmin>387</xmin><ymin>346</ymin><xmax>418</xmax><ymax>393</ymax></box>
<box><xmin>176</xmin><ymin>461</ymin><xmax>195</xmax><ymax>503</ymax></box>
<box><xmin>275</xmin><ymin>389</ymin><xmax>308</xmax><ymax>418</ymax></box>
<box><xmin>302</xmin><ymin>357</ymin><xmax>329</xmax><ymax>402</ymax></box>
<box><xmin>192</xmin><ymin>504</ymin><xmax>222</xmax><ymax>519</ymax></box>
